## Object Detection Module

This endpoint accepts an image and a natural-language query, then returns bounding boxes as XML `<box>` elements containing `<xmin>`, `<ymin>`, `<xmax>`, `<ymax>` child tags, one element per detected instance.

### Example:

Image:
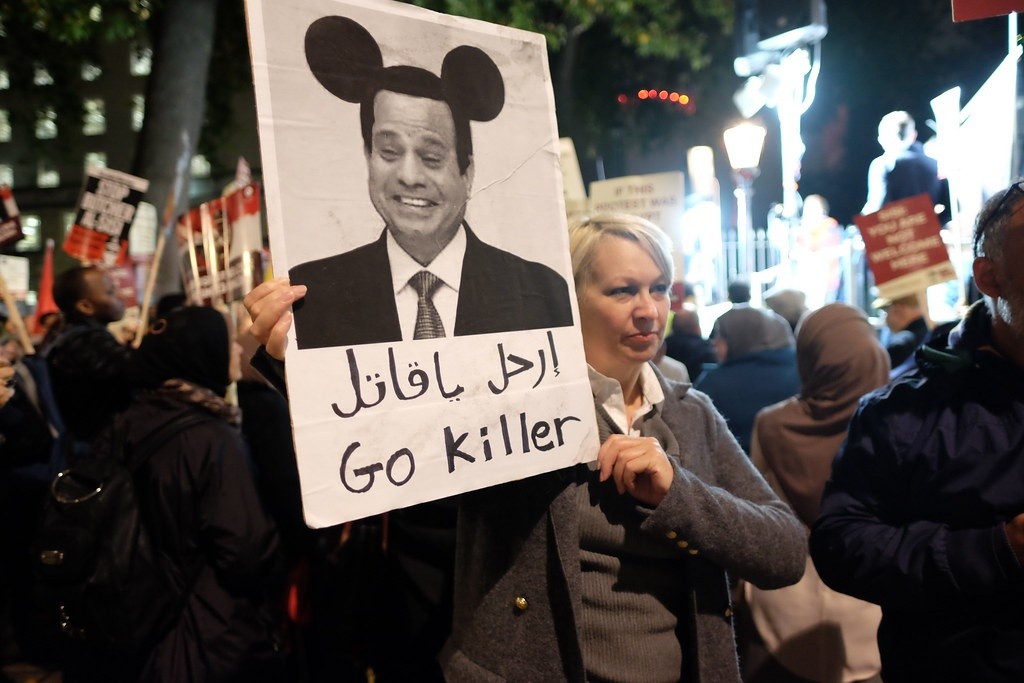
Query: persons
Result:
<box><xmin>243</xmin><ymin>211</ymin><xmax>808</xmax><ymax>683</ymax></box>
<box><xmin>1</xmin><ymin>290</ymin><xmax>149</xmax><ymax>669</ymax></box>
<box><xmin>668</xmin><ymin>280</ymin><xmax>936</xmax><ymax>460</ymax></box>
<box><xmin>36</xmin><ymin>266</ymin><xmax>141</xmax><ymax>449</ymax></box>
<box><xmin>287</xmin><ymin>16</ymin><xmax>574</xmax><ymax>351</ymax></box>
<box><xmin>787</xmin><ymin>194</ymin><xmax>844</xmax><ymax>313</ymax></box>
<box><xmin>96</xmin><ymin>304</ymin><xmax>273</xmax><ymax>683</ymax></box>
<box><xmin>860</xmin><ymin>111</ymin><xmax>940</xmax><ymax>217</ymax></box>
<box><xmin>744</xmin><ymin>301</ymin><xmax>892</xmax><ymax>682</ymax></box>
<box><xmin>807</xmin><ymin>181</ymin><xmax>1024</xmax><ymax>682</ymax></box>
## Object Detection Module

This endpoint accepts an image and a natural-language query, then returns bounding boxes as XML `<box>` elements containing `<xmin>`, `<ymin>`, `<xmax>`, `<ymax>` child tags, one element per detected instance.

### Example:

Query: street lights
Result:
<box><xmin>714</xmin><ymin>114</ymin><xmax>771</xmax><ymax>298</ymax></box>
<box><xmin>674</xmin><ymin>140</ymin><xmax>728</xmax><ymax>314</ymax></box>
<box><xmin>736</xmin><ymin>22</ymin><xmax>833</xmax><ymax>300</ymax></box>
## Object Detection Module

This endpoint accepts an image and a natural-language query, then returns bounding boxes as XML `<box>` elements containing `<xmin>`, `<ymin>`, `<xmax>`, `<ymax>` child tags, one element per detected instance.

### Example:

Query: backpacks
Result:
<box><xmin>12</xmin><ymin>409</ymin><xmax>218</xmax><ymax>673</ymax></box>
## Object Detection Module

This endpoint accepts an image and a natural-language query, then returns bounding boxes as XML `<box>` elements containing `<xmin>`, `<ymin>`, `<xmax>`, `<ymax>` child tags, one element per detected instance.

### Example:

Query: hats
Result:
<box><xmin>871</xmin><ymin>293</ymin><xmax>918</xmax><ymax>309</ymax></box>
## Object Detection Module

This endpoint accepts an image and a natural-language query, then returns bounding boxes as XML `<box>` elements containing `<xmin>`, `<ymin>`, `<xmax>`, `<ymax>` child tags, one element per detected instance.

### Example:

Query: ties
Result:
<box><xmin>407</xmin><ymin>270</ymin><xmax>446</xmax><ymax>340</ymax></box>
